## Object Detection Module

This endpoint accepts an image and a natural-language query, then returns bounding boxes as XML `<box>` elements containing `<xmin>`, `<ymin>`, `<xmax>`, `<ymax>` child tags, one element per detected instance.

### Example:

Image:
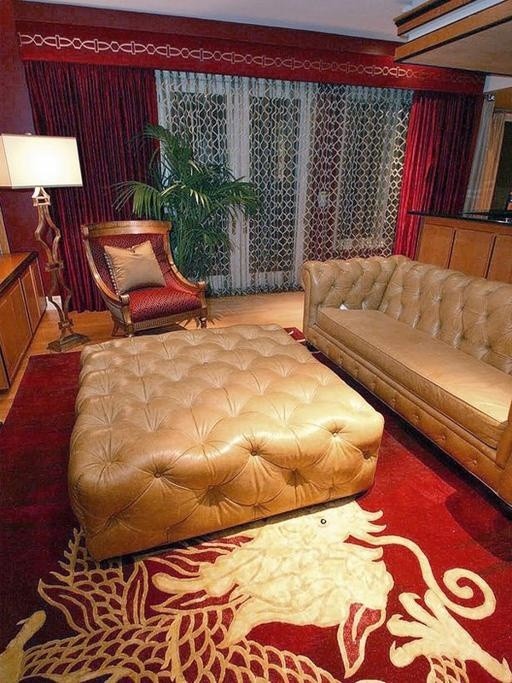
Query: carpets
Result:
<box><xmin>1</xmin><ymin>324</ymin><xmax>512</xmax><ymax>681</ymax></box>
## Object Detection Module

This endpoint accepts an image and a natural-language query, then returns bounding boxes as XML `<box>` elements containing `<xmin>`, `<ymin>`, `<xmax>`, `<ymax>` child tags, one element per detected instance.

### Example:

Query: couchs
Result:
<box><xmin>302</xmin><ymin>253</ymin><xmax>512</xmax><ymax>510</ymax></box>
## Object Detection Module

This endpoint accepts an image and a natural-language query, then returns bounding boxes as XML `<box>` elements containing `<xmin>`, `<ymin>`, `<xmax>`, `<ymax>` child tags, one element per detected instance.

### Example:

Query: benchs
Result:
<box><xmin>66</xmin><ymin>323</ymin><xmax>385</xmax><ymax>562</ymax></box>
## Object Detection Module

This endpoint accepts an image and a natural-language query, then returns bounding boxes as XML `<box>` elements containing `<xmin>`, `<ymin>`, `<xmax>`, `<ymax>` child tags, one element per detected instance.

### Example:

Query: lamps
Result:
<box><xmin>0</xmin><ymin>130</ymin><xmax>91</xmax><ymax>352</ymax></box>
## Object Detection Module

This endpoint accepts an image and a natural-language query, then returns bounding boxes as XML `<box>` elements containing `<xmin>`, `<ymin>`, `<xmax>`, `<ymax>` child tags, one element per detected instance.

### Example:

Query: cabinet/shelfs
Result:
<box><xmin>414</xmin><ymin>218</ymin><xmax>512</xmax><ymax>286</ymax></box>
<box><xmin>0</xmin><ymin>251</ymin><xmax>47</xmax><ymax>392</ymax></box>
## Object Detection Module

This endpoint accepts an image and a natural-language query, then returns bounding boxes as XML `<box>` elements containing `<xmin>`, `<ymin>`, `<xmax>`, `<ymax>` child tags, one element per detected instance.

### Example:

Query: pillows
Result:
<box><xmin>103</xmin><ymin>240</ymin><xmax>166</xmax><ymax>297</ymax></box>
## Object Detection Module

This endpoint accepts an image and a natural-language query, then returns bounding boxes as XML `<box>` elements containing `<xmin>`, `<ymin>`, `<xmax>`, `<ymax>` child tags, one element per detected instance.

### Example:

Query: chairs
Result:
<box><xmin>80</xmin><ymin>218</ymin><xmax>208</xmax><ymax>339</ymax></box>
